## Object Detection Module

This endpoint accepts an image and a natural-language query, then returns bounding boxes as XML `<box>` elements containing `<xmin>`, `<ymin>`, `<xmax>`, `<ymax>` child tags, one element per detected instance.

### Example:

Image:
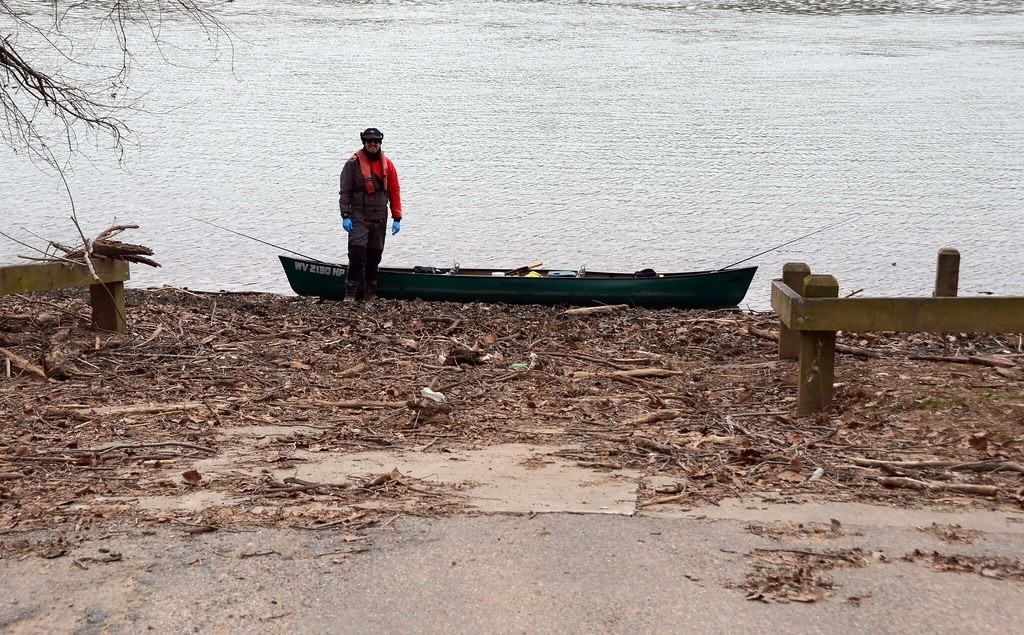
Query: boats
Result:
<box><xmin>278</xmin><ymin>254</ymin><xmax>758</xmax><ymax>309</ymax></box>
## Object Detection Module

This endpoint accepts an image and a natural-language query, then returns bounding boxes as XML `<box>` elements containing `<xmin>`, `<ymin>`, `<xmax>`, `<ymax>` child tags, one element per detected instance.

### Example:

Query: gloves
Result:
<box><xmin>392</xmin><ymin>220</ymin><xmax>400</xmax><ymax>235</ymax></box>
<box><xmin>343</xmin><ymin>218</ymin><xmax>353</xmax><ymax>232</ymax></box>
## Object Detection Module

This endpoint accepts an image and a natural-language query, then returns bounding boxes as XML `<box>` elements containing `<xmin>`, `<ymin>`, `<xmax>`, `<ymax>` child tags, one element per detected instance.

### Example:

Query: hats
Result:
<box><xmin>360</xmin><ymin>128</ymin><xmax>384</xmax><ymax>140</ymax></box>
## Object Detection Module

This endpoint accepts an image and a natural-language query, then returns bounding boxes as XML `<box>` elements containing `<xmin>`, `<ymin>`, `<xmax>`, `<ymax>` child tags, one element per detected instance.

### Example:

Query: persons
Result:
<box><xmin>339</xmin><ymin>128</ymin><xmax>403</xmax><ymax>303</ymax></box>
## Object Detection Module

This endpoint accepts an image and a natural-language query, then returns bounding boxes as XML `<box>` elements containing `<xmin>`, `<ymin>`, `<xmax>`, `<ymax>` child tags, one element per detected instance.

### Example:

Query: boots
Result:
<box><xmin>343</xmin><ymin>280</ymin><xmax>359</xmax><ymax>302</ymax></box>
<box><xmin>364</xmin><ymin>280</ymin><xmax>377</xmax><ymax>301</ymax></box>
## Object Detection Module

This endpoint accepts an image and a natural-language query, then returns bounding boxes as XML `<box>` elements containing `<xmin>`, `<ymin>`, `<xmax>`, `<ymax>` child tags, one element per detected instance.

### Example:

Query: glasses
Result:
<box><xmin>365</xmin><ymin>140</ymin><xmax>380</xmax><ymax>144</ymax></box>
